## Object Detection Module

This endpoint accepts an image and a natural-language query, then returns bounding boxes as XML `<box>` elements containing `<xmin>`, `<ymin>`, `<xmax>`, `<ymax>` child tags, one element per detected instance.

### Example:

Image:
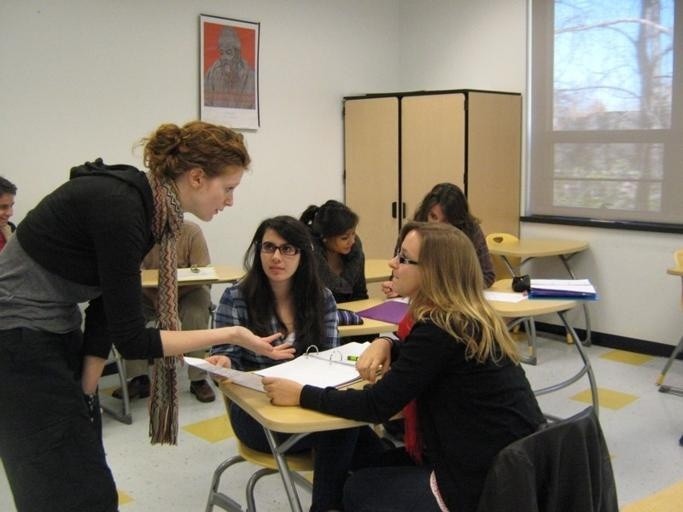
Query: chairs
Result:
<box><xmin>205</xmin><ymin>392</ymin><xmax>316</xmax><ymax>512</ymax></box>
<box><xmin>656</xmin><ymin>251</ymin><xmax>683</xmax><ymax>386</ymax></box>
<box><xmin>485</xmin><ymin>233</ymin><xmax>573</xmax><ymax>354</ymax></box>
<box><xmin>479</xmin><ymin>405</ymin><xmax>619</xmax><ymax>512</ymax></box>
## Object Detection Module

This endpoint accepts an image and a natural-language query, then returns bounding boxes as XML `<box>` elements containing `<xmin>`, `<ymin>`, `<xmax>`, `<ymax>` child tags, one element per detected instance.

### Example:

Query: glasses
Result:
<box><xmin>260</xmin><ymin>241</ymin><xmax>301</xmax><ymax>256</ymax></box>
<box><xmin>396</xmin><ymin>247</ymin><xmax>418</xmax><ymax>265</ymax></box>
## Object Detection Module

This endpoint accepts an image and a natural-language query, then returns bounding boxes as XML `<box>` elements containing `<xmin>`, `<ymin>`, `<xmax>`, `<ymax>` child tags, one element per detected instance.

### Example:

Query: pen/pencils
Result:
<box><xmin>348</xmin><ymin>355</ymin><xmax>360</xmax><ymax>361</ymax></box>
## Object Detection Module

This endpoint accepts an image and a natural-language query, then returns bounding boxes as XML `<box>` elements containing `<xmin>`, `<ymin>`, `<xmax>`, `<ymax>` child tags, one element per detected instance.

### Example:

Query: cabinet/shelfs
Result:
<box><xmin>342</xmin><ymin>90</ymin><xmax>522</xmax><ymax>281</ymax></box>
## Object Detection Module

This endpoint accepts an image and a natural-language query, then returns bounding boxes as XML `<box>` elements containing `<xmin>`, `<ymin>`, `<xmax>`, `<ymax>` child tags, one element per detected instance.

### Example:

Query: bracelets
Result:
<box><xmin>373</xmin><ymin>337</ymin><xmax>393</xmax><ymax>349</ymax></box>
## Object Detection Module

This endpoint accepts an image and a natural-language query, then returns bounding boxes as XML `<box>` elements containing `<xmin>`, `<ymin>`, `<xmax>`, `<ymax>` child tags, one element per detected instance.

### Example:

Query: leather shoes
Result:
<box><xmin>112</xmin><ymin>378</ymin><xmax>150</xmax><ymax>399</ymax></box>
<box><xmin>190</xmin><ymin>379</ymin><xmax>215</xmax><ymax>402</ymax></box>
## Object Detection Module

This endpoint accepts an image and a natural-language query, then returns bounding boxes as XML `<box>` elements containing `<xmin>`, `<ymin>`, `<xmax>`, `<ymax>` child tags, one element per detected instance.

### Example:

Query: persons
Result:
<box><xmin>203</xmin><ymin>213</ymin><xmax>378</xmax><ymax>511</ymax></box>
<box><xmin>262</xmin><ymin>220</ymin><xmax>548</xmax><ymax>512</ymax></box>
<box><xmin>298</xmin><ymin>199</ymin><xmax>381</xmax><ymax>345</ymax></box>
<box><xmin>381</xmin><ymin>183</ymin><xmax>495</xmax><ymax>300</ymax></box>
<box><xmin>0</xmin><ymin>176</ymin><xmax>16</xmax><ymax>252</ymax></box>
<box><xmin>204</xmin><ymin>27</ymin><xmax>255</xmax><ymax>110</ymax></box>
<box><xmin>111</xmin><ymin>218</ymin><xmax>215</xmax><ymax>403</ymax></box>
<box><xmin>0</xmin><ymin>120</ymin><xmax>296</xmax><ymax>511</ymax></box>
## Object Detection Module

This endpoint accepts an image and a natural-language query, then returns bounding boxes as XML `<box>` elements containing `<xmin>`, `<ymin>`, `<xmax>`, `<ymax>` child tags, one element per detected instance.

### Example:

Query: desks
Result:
<box><xmin>489</xmin><ymin>240</ymin><xmax>591</xmax><ymax>365</ymax></box>
<box><xmin>218</xmin><ymin>360</ymin><xmax>404</xmax><ymax>512</ymax></box>
<box><xmin>99</xmin><ymin>267</ymin><xmax>248</xmax><ymax>424</ymax></box>
<box><xmin>667</xmin><ymin>265</ymin><xmax>683</xmax><ymax>276</ymax></box>
<box><xmin>336</xmin><ymin>299</ymin><xmax>400</xmax><ymax>336</ymax></box>
<box><xmin>364</xmin><ymin>259</ymin><xmax>396</xmax><ymax>283</ymax></box>
<box><xmin>485</xmin><ymin>279</ymin><xmax>599</xmax><ymax>422</ymax></box>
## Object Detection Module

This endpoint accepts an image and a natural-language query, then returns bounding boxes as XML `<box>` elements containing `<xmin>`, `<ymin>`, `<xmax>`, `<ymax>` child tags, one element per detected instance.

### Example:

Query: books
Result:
<box><xmin>246</xmin><ymin>339</ymin><xmax>385</xmax><ymax>390</ymax></box>
